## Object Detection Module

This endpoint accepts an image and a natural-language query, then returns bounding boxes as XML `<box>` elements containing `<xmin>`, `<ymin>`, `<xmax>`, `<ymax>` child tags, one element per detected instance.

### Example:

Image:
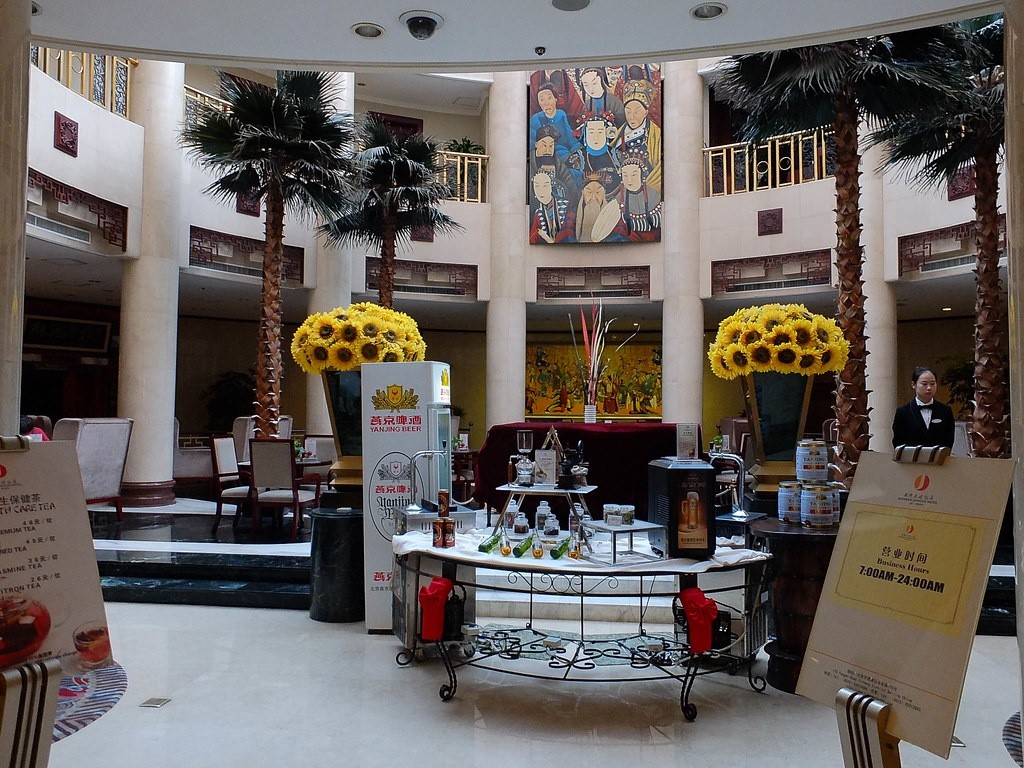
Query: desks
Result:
<box><xmin>235</xmin><ymin>457</ymin><xmax>332</xmax><ymax>533</ymax></box>
<box><xmin>473</xmin><ymin>418</ymin><xmax>702</xmax><ymax>521</ymax></box>
<box><xmin>721</xmin><ymin>511</ymin><xmax>841</xmax><ymax>694</ymax></box>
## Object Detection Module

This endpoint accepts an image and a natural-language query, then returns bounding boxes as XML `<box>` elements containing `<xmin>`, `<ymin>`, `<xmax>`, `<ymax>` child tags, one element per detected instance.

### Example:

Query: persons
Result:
<box><xmin>20</xmin><ymin>415</ymin><xmax>50</xmax><ymax>441</ymax></box>
<box><xmin>892</xmin><ymin>366</ymin><xmax>955</xmax><ymax>456</ymax></box>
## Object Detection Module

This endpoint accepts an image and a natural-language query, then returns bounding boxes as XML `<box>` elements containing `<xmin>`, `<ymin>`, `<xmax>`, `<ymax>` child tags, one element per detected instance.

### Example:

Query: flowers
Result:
<box><xmin>290</xmin><ymin>300</ymin><xmax>427</xmax><ymax>374</ymax></box>
<box><xmin>707</xmin><ymin>303</ymin><xmax>850</xmax><ymax>381</ymax></box>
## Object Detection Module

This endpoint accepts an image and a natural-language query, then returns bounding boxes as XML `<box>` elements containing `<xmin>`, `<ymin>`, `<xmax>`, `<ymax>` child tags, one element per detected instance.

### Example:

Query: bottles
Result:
<box><xmin>550</xmin><ymin>503</ymin><xmax>596</xmax><ymax>559</ymax></box>
<box><xmin>513</xmin><ymin>513</ymin><xmax>530</xmax><ymax>534</ymax></box>
<box><xmin>543</xmin><ymin>514</ymin><xmax>560</xmax><ymax>537</ymax></box>
<box><xmin>500</xmin><ymin>526</ymin><xmax>511</xmax><ymax>555</ymax></box>
<box><xmin>604</xmin><ymin>504</ymin><xmax>635</xmax><ymax>525</ymax></box>
<box><xmin>535</xmin><ymin>501</ymin><xmax>551</xmax><ymax>532</ymax></box>
<box><xmin>504</xmin><ymin>501</ymin><xmax>519</xmax><ymax>530</ymax></box>
<box><xmin>532</xmin><ymin>528</ymin><xmax>543</xmax><ymax>557</ymax></box>
<box><xmin>478</xmin><ymin>532</ymin><xmax>500</xmax><ymax>553</ymax></box>
<box><xmin>512</xmin><ymin>535</ymin><xmax>532</xmax><ymax>557</ymax></box>
<box><xmin>688</xmin><ymin>495</ymin><xmax>698</xmax><ymax>529</ymax></box>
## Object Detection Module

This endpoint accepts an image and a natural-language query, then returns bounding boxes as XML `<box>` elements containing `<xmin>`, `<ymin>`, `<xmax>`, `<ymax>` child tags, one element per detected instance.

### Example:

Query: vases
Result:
<box><xmin>319</xmin><ymin>369</ymin><xmax>364</xmax><ymax>496</ymax></box>
<box><xmin>742</xmin><ymin>368</ymin><xmax>815</xmax><ymax>513</ymax></box>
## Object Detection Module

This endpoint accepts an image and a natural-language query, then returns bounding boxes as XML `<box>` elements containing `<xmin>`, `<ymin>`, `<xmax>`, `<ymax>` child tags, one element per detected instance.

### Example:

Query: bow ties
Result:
<box><xmin>918</xmin><ymin>404</ymin><xmax>933</xmax><ymax>410</ymax></box>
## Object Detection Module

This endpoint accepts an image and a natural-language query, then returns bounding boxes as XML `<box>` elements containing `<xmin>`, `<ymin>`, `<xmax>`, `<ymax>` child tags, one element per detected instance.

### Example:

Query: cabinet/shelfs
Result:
<box><xmin>417</xmin><ymin>530</ymin><xmax>772</xmax><ymax>722</ymax></box>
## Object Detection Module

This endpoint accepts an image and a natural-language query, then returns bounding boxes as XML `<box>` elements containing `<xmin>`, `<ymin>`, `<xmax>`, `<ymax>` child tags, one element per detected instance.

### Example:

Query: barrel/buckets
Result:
<box><xmin>777</xmin><ymin>441</ymin><xmax>840</xmax><ymax>526</ymax></box>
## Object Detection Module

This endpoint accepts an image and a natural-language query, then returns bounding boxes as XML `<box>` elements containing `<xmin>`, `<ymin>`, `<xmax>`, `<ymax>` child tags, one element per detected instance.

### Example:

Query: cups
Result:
<box><xmin>72</xmin><ymin>620</ymin><xmax>113</xmax><ymax>671</ymax></box>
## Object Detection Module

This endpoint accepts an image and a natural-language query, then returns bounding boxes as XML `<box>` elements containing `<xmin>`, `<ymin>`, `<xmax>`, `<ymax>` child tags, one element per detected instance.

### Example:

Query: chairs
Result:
<box><xmin>53</xmin><ymin>416</ymin><xmax>134</xmax><ymax>522</ymax></box>
<box><xmin>210</xmin><ymin>431</ymin><xmax>338</xmax><ymax>544</ymax></box>
